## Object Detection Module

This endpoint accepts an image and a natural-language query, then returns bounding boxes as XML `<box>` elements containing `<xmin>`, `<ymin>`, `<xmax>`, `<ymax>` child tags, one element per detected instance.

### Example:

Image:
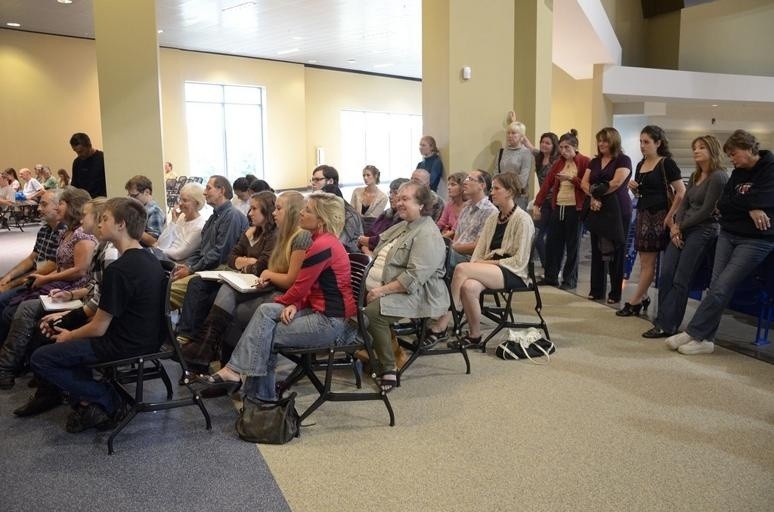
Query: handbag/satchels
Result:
<box><xmin>496</xmin><ymin>327</ymin><xmax>555</xmax><ymax>365</ymax></box>
<box><xmin>661</xmin><ymin>157</ymin><xmax>676</xmax><ymax>211</ymax></box>
<box><xmin>236</xmin><ymin>391</ymin><xmax>300</xmax><ymax>444</ymax></box>
<box><xmin>354</xmin><ymin>330</ymin><xmax>407</xmax><ymax>376</ymax></box>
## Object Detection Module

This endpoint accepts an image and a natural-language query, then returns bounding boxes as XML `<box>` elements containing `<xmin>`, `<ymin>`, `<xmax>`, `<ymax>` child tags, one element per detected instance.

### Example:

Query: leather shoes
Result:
<box><xmin>14</xmin><ymin>390</ymin><xmax>64</xmax><ymax>416</ymax></box>
<box><xmin>642</xmin><ymin>325</ymin><xmax>677</xmax><ymax>338</ymax></box>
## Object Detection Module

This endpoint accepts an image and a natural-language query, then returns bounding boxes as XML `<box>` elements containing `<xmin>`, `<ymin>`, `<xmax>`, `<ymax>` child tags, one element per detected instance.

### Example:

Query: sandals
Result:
<box><xmin>447</xmin><ymin>331</ymin><xmax>483</xmax><ymax>350</ymax></box>
<box><xmin>380</xmin><ymin>371</ymin><xmax>396</xmax><ymax>391</ymax></box>
<box><xmin>608</xmin><ymin>295</ymin><xmax>620</xmax><ymax>303</ymax></box>
<box><xmin>588</xmin><ymin>293</ymin><xmax>606</xmax><ymax>299</ymax></box>
<box><xmin>422</xmin><ymin>326</ymin><xmax>449</xmax><ymax>348</ymax></box>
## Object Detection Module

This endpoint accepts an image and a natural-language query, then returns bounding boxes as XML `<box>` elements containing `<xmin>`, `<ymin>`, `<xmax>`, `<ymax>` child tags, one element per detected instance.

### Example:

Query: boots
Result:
<box><xmin>0</xmin><ymin>320</ymin><xmax>37</xmax><ymax>391</ymax></box>
<box><xmin>170</xmin><ymin>304</ymin><xmax>233</xmax><ymax>366</ymax></box>
<box><xmin>200</xmin><ymin>340</ymin><xmax>242</xmax><ymax>397</ymax></box>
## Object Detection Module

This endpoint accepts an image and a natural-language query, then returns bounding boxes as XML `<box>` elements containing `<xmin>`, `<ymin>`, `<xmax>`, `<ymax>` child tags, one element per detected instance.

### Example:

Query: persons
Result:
<box><xmin>413</xmin><ymin>135</ymin><xmax>443</xmax><ymax>192</ymax></box>
<box><xmin>1</xmin><ymin>160</ymin><xmax>556</xmax><ymax>432</ymax></box>
<box><xmin>358</xmin><ymin>178</ymin><xmax>450</xmax><ymax>396</ymax></box>
<box><xmin>616</xmin><ymin>127</ymin><xmax>687</xmax><ymax>316</ymax></box>
<box><xmin>63</xmin><ymin>131</ymin><xmax>104</xmax><ymax>199</ymax></box>
<box><xmin>664</xmin><ymin>127</ymin><xmax>774</xmax><ymax>355</ymax></box>
<box><xmin>21</xmin><ymin>194</ymin><xmax>170</xmax><ymax>427</ymax></box>
<box><xmin>194</xmin><ymin>187</ymin><xmax>356</xmax><ymax>416</ymax></box>
<box><xmin>490</xmin><ymin>110</ymin><xmax>632</xmax><ymax>303</ymax></box>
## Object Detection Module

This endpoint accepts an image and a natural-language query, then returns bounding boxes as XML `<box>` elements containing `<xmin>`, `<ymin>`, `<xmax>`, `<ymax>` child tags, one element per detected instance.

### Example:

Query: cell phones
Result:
<box><xmin>556</xmin><ymin>173</ymin><xmax>565</xmax><ymax>177</ymax></box>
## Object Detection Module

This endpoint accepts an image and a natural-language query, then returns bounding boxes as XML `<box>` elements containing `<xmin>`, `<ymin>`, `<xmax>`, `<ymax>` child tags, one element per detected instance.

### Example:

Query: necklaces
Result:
<box><xmin>639</xmin><ymin>153</ymin><xmax>658</xmax><ymax>184</ymax></box>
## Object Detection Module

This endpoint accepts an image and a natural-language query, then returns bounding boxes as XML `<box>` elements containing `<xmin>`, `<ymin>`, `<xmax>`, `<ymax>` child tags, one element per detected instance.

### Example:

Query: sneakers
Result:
<box><xmin>66</xmin><ymin>405</ymin><xmax>106</xmax><ymax>433</ymax></box>
<box><xmin>678</xmin><ymin>339</ymin><xmax>715</xmax><ymax>354</ymax></box>
<box><xmin>665</xmin><ymin>331</ymin><xmax>691</xmax><ymax>349</ymax></box>
<box><xmin>537</xmin><ymin>278</ymin><xmax>559</xmax><ymax>286</ymax></box>
<box><xmin>559</xmin><ymin>281</ymin><xmax>576</xmax><ymax>289</ymax></box>
<box><xmin>96</xmin><ymin>399</ymin><xmax>128</xmax><ymax>430</ymax></box>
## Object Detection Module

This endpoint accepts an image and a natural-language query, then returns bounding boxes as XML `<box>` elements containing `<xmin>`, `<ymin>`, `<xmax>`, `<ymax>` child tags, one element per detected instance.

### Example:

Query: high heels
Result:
<box><xmin>195</xmin><ymin>373</ymin><xmax>241</xmax><ymax>396</ymax></box>
<box><xmin>616</xmin><ymin>302</ymin><xmax>642</xmax><ymax>316</ymax></box>
<box><xmin>642</xmin><ymin>297</ymin><xmax>651</xmax><ymax>311</ymax></box>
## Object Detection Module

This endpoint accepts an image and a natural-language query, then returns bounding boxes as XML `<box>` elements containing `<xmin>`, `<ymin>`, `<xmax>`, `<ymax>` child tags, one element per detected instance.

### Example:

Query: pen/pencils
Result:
<box><xmin>48</xmin><ymin>285</ymin><xmax>71</xmax><ymax>298</ymax></box>
<box><xmin>251</xmin><ymin>278</ymin><xmax>270</xmax><ymax>288</ymax></box>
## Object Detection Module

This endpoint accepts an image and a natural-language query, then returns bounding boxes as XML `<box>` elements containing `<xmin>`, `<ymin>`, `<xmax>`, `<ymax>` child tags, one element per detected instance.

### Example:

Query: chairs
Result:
<box><xmin>164</xmin><ymin>173</ymin><xmax>206</xmax><ymax>204</ymax></box>
<box><xmin>0</xmin><ymin>193</ymin><xmax>39</xmax><ymax>230</ymax></box>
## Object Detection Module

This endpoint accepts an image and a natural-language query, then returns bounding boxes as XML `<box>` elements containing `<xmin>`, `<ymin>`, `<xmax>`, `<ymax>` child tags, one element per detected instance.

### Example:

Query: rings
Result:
<box><xmin>758</xmin><ymin>219</ymin><xmax>762</xmax><ymax>223</ymax></box>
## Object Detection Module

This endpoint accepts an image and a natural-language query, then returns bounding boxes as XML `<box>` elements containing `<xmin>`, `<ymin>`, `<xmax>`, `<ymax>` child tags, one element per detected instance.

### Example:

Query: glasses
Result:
<box><xmin>129</xmin><ymin>192</ymin><xmax>142</xmax><ymax>198</ymax></box>
<box><xmin>310</xmin><ymin>178</ymin><xmax>326</xmax><ymax>183</ymax></box>
<box><xmin>465</xmin><ymin>175</ymin><xmax>481</xmax><ymax>184</ymax></box>
